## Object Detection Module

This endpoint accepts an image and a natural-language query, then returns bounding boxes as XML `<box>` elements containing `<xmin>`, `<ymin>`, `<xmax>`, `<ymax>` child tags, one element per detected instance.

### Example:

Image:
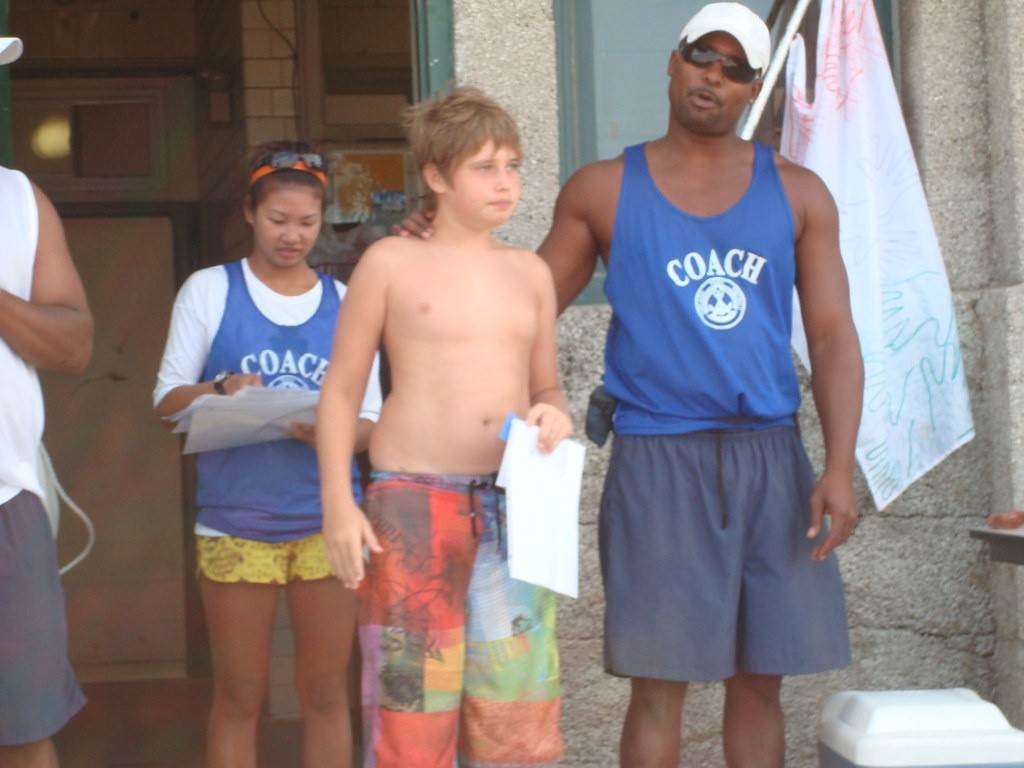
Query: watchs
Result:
<box><xmin>212</xmin><ymin>370</ymin><xmax>235</xmax><ymax>396</ymax></box>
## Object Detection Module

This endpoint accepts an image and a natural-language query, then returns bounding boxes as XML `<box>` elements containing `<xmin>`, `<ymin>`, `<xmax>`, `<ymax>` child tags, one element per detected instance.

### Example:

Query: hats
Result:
<box><xmin>0</xmin><ymin>36</ymin><xmax>24</xmax><ymax>65</ymax></box>
<box><xmin>678</xmin><ymin>3</ymin><xmax>771</xmax><ymax>78</ymax></box>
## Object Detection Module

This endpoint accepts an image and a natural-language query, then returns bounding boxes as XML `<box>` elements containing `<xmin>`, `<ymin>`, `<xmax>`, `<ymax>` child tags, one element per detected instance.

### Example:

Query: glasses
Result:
<box><xmin>683</xmin><ymin>44</ymin><xmax>755</xmax><ymax>83</ymax></box>
<box><xmin>254</xmin><ymin>151</ymin><xmax>328</xmax><ymax>175</ymax></box>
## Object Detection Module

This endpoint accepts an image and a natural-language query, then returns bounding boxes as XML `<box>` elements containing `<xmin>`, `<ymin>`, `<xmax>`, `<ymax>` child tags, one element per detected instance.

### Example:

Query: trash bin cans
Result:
<box><xmin>816</xmin><ymin>687</ymin><xmax>1024</xmax><ymax>768</ymax></box>
<box><xmin>967</xmin><ymin>527</ymin><xmax>1023</xmax><ymax>731</ymax></box>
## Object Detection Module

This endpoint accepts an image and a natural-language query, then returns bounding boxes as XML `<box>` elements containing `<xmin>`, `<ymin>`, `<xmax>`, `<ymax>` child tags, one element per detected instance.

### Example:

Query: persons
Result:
<box><xmin>390</xmin><ymin>0</ymin><xmax>865</xmax><ymax>768</ymax></box>
<box><xmin>316</xmin><ymin>87</ymin><xmax>574</xmax><ymax>768</ymax></box>
<box><xmin>153</xmin><ymin>141</ymin><xmax>383</xmax><ymax>768</ymax></box>
<box><xmin>0</xmin><ymin>36</ymin><xmax>95</xmax><ymax>768</ymax></box>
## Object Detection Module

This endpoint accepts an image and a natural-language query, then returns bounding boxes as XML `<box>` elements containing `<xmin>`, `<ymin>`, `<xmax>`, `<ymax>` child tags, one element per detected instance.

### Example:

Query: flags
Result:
<box><xmin>778</xmin><ymin>0</ymin><xmax>977</xmax><ymax>514</ymax></box>
